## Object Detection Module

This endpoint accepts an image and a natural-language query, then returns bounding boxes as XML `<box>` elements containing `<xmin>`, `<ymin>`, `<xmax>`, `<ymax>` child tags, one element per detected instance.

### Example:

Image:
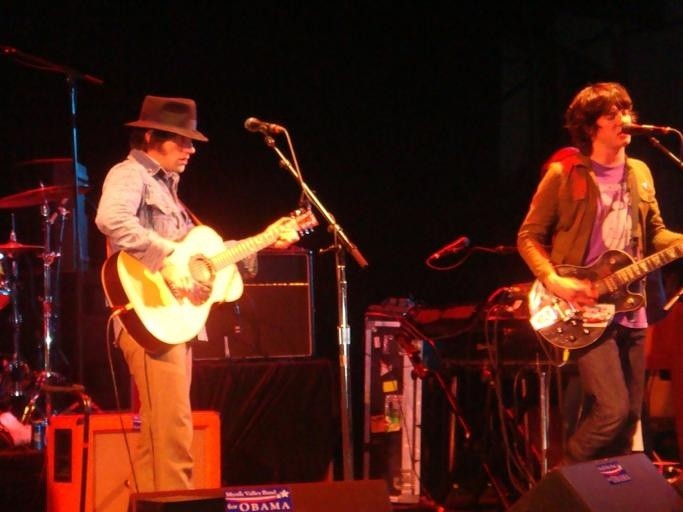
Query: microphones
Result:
<box><xmin>243</xmin><ymin>117</ymin><xmax>281</xmax><ymax>137</ymax></box>
<box><xmin>428</xmin><ymin>235</ymin><xmax>471</xmax><ymax>262</ymax></box>
<box><xmin>621</xmin><ymin>123</ymin><xmax>669</xmax><ymax>137</ymax></box>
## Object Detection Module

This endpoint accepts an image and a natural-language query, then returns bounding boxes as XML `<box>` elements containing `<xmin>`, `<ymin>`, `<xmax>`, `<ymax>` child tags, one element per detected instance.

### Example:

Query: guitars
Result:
<box><xmin>525</xmin><ymin>235</ymin><xmax>683</xmax><ymax>349</ymax></box>
<box><xmin>101</xmin><ymin>204</ymin><xmax>323</xmax><ymax>356</ymax></box>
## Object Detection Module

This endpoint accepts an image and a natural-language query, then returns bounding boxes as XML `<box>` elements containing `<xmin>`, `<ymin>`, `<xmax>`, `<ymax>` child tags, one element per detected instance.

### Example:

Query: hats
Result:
<box><xmin>122</xmin><ymin>95</ymin><xmax>210</xmax><ymax>144</ymax></box>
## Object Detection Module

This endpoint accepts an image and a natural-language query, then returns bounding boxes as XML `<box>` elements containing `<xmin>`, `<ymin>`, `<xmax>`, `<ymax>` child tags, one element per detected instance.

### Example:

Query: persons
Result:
<box><xmin>95</xmin><ymin>95</ymin><xmax>299</xmax><ymax>494</ymax></box>
<box><xmin>515</xmin><ymin>80</ymin><xmax>682</xmax><ymax>463</ymax></box>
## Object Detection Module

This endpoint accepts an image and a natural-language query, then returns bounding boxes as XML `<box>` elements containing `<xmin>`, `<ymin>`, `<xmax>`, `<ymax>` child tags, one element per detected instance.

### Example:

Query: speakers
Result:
<box><xmin>192</xmin><ymin>253</ymin><xmax>316</xmax><ymax>362</ymax></box>
<box><xmin>506</xmin><ymin>452</ymin><xmax>683</xmax><ymax>512</ymax></box>
<box><xmin>128</xmin><ymin>478</ymin><xmax>391</xmax><ymax>512</ymax></box>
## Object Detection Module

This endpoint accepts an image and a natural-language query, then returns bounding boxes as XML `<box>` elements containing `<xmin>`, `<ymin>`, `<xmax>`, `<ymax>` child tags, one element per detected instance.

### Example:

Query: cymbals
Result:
<box><xmin>2</xmin><ymin>235</ymin><xmax>45</xmax><ymax>257</ymax></box>
<box><xmin>0</xmin><ymin>184</ymin><xmax>93</xmax><ymax>213</ymax></box>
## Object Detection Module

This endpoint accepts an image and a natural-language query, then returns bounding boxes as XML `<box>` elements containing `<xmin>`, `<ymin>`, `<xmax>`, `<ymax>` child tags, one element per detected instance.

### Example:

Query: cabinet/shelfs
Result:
<box><xmin>45</xmin><ymin>411</ymin><xmax>222</xmax><ymax>512</ymax></box>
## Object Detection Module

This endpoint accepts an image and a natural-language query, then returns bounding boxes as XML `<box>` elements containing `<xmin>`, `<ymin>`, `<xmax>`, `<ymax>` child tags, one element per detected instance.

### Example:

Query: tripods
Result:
<box><xmin>20</xmin><ymin>218</ymin><xmax>102</xmax><ymax>424</ymax></box>
